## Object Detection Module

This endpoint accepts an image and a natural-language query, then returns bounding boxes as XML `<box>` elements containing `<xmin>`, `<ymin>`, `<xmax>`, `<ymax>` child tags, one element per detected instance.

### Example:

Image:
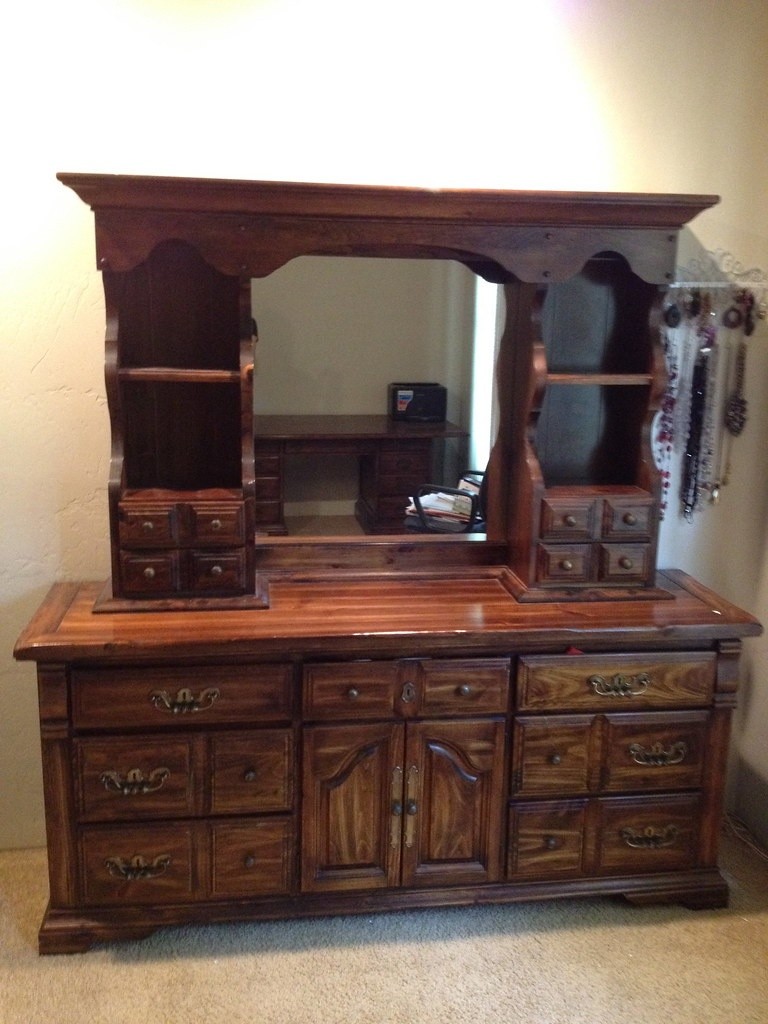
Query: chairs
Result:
<box><xmin>403</xmin><ymin>470</ymin><xmax>485</xmax><ymax>534</ymax></box>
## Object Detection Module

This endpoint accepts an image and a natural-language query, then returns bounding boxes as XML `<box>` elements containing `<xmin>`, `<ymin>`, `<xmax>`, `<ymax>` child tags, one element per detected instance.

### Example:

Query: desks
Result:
<box><xmin>254</xmin><ymin>416</ymin><xmax>471</xmax><ymax>538</ymax></box>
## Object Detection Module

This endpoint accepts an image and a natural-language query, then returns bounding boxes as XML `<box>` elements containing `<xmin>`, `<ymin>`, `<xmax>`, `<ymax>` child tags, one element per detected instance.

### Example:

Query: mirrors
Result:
<box><xmin>246</xmin><ymin>246</ymin><xmax>510</xmax><ymax>549</ymax></box>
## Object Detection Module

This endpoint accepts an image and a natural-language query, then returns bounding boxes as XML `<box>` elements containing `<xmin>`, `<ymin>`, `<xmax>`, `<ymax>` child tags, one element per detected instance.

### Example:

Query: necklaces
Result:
<box><xmin>657</xmin><ymin>325</ymin><xmax>746</xmax><ymax>520</ymax></box>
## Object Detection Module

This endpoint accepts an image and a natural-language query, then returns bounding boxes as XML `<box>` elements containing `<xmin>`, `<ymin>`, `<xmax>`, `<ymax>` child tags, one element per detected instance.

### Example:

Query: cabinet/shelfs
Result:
<box><xmin>13</xmin><ymin>569</ymin><xmax>766</xmax><ymax>958</ymax></box>
<box><xmin>57</xmin><ymin>172</ymin><xmax>722</xmax><ymax>610</ymax></box>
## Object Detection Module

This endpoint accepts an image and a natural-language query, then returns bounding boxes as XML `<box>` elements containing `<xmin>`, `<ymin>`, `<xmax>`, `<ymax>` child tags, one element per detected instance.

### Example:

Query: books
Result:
<box><xmin>407</xmin><ymin>493</ymin><xmax>472</xmax><ymax>523</ymax></box>
